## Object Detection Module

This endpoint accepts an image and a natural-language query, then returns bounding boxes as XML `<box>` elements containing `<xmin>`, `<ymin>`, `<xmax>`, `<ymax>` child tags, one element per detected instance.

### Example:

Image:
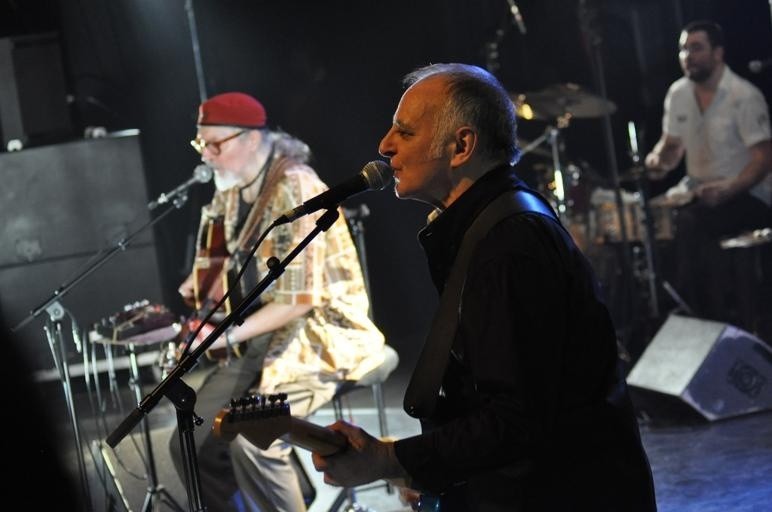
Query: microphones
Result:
<box><xmin>144</xmin><ymin>164</ymin><xmax>213</xmax><ymax>211</ymax></box>
<box><xmin>274</xmin><ymin>159</ymin><xmax>394</xmax><ymax>226</ymax></box>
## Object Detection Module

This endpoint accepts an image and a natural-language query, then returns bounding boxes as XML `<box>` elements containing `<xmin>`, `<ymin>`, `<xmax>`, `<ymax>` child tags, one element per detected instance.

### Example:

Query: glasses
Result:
<box><xmin>188</xmin><ymin>127</ymin><xmax>250</xmax><ymax>157</ymax></box>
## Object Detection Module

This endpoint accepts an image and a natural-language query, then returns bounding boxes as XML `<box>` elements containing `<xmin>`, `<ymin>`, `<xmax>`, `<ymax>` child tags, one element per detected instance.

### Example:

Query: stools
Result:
<box><xmin>320</xmin><ymin>346</ymin><xmax>404</xmax><ymax>512</ymax></box>
<box><xmin>88</xmin><ymin>324</ymin><xmax>186</xmax><ymax>512</ymax></box>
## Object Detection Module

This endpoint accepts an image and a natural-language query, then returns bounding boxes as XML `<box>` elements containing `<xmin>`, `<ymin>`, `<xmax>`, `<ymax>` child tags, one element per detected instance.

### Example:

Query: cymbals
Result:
<box><xmin>508</xmin><ymin>85</ymin><xmax>617</xmax><ymax>121</ymax></box>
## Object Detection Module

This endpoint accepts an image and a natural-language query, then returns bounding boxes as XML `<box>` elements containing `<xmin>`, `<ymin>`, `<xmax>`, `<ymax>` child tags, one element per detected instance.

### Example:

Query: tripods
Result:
<box><xmin>127</xmin><ymin>342</ymin><xmax>184</xmax><ymax>512</ymax></box>
<box><xmin>619</xmin><ymin>170</ymin><xmax>696</xmax><ymax>327</ymax></box>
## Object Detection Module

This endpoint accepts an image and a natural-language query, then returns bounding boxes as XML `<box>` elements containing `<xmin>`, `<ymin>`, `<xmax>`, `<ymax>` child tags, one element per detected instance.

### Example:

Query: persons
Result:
<box><xmin>177</xmin><ymin>92</ymin><xmax>401</xmax><ymax>511</ymax></box>
<box><xmin>309</xmin><ymin>64</ymin><xmax>655</xmax><ymax>512</ymax></box>
<box><xmin>638</xmin><ymin>16</ymin><xmax>769</xmax><ymax>317</ymax></box>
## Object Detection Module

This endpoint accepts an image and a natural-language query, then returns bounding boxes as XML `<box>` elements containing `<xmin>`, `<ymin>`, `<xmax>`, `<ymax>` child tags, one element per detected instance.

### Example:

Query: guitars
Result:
<box><xmin>212</xmin><ymin>393</ymin><xmax>451</xmax><ymax>511</ymax></box>
<box><xmin>161</xmin><ymin>216</ymin><xmax>244</xmax><ymax>382</ymax></box>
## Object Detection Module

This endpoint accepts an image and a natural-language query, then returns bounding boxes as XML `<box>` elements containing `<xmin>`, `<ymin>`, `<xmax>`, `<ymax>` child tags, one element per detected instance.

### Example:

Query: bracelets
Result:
<box><xmin>226</xmin><ymin>326</ymin><xmax>240</xmax><ymax>349</ymax></box>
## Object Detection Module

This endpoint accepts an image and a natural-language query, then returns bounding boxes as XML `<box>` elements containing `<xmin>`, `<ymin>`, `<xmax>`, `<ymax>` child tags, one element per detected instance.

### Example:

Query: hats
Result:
<box><xmin>194</xmin><ymin>89</ymin><xmax>269</xmax><ymax>131</ymax></box>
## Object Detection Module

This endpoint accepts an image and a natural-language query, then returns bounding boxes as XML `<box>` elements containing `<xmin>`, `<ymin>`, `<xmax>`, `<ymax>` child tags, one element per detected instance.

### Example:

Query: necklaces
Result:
<box><xmin>238</xmin><ymin>155</ymin><xmax>274</xmax><ymax>192</ymax></box>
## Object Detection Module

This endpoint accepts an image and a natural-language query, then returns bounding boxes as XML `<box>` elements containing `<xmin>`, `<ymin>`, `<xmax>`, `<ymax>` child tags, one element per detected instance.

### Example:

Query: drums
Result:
<box><xmin>541</xmin><ymin>167</ymin><xmax>595</xmax><ymax>221</ymax></box>
<box><xmin>593</xmin><ymin>191</ymin><xmax>697</xmax><ymax>244</ymax></box>
<box><xmin>719</xmin><ymin>229</ymin><xmax>772</xmax><ymax>313</ymax></box>
